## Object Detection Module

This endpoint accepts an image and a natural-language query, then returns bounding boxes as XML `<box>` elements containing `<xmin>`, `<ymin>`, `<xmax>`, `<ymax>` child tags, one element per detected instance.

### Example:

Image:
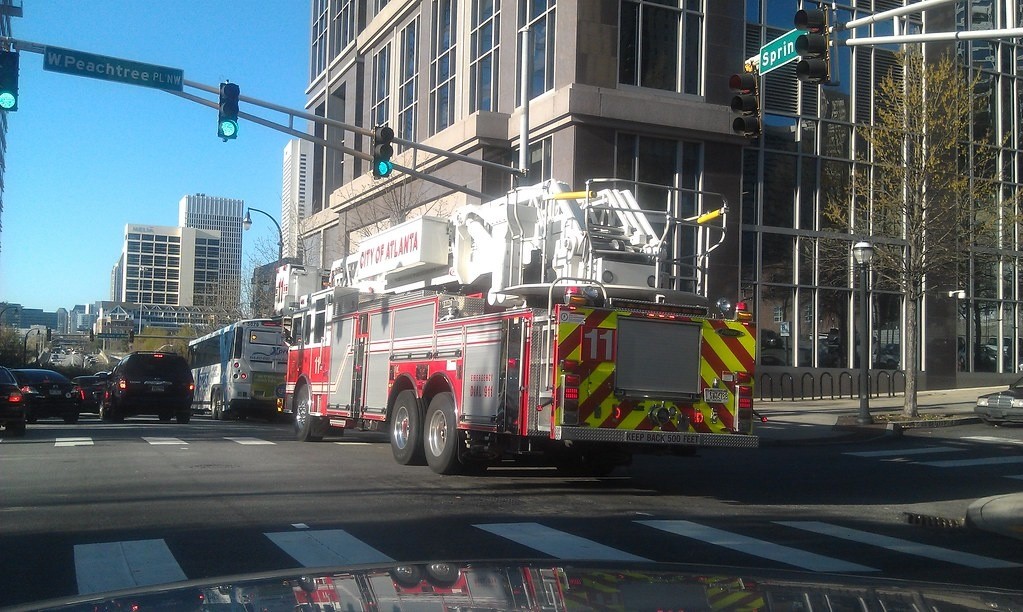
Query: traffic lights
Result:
<box><xmin>727</xmin><ymin>65</ymin><xmax>763</xmax><ymax>139</ymax></box>
<box><xmin>217</xmin><ymin>82</ymin><xmax>240</xmax><ymax>140</ymax></box>
<box><xmin>373</xmin><ymin>126</ymin><xmax>394</xmax><ymax>178</ymax></box>
<box><xmin>0</xmin><ymin>51</ymin><xmax>19</xmax><ymax>112</ymax></box>
<box><xmin>794</xmin><ymin>7</ymin><xmax>831</xmax><ymax>85</ymax></box>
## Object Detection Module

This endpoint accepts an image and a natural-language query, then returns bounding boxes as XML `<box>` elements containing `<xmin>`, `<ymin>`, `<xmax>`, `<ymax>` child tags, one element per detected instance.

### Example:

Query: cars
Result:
<box><xmin>0</xmin><ymin>365</ymin><xmax>108</xmax><ymax>436</ymax></box>
<box><xmin>752</xmin><ymin>327</ymin><xmax>1023</xmax><ymax>377</ymax></box>
<box><xmin>973</xmin><ymin>376</ymin><xmax>1023</xmax><ymax>426</ymax></box>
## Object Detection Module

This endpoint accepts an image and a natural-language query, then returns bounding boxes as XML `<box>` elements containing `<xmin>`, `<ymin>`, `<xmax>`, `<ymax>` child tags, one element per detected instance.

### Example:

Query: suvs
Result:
<box><xmin>99</xmin><ymin>349</ymin><xmax>196</xmax><ymax>423</ymax></box>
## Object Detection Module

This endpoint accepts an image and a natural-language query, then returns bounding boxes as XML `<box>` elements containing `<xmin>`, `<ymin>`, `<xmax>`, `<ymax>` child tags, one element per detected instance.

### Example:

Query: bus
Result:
<box><xmin>185</xmin><ymin>316</ymin><xmax>304</xmax><ymax>421</ymax></box>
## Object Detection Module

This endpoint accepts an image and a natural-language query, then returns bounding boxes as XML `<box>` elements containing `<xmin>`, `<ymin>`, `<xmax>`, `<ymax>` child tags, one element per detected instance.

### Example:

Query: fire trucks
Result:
<box><xmin>277</xmin><ymin>176</ymin><xmax>763</xmax><ymax>476</ymax></box>
<box><xmin>277</xmin><ymin>562</ymin><xmax>765</xmax><ymax>612</ymax></box>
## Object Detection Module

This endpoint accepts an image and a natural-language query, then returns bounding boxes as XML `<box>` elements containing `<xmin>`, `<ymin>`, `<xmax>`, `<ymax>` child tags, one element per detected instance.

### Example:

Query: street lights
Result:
<box><xmin>24</xmin><ymin>328</ymin><xmax>41</xmax><ymax>365</ymax></box>
<box><xmin>242</xmin><ymin>206</ymin><xmax>285</xmax><ymax>260</ymax></box>
<box><xmin>852</xmin><ymin>241</ymin><xmax>876</xmax><ymax>425</ymax></box>
